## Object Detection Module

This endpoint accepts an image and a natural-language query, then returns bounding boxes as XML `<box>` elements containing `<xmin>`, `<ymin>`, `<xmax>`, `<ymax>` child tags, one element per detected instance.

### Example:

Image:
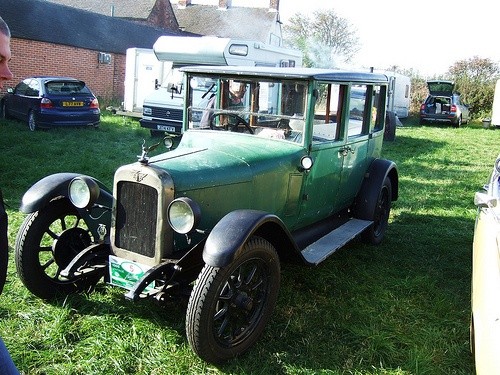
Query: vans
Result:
<box><xmin>16</xmin><ymin>68</ymin><xmax>398</xmax><ymax>364</ymax></box>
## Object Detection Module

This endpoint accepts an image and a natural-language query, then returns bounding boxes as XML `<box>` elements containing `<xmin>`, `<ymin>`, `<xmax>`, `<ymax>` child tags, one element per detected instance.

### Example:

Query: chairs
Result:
<box><xmin>241</xmin><ymin>120</ymin><xmax>328</xmax><ymax>144</ymax></box>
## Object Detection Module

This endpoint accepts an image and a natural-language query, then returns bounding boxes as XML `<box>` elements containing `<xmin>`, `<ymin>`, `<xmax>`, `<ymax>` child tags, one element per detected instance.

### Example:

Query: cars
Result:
<box><xmin>421</xmin><ymin>81</ymin><xmax>469</xmax><ymax>125</ymax></box>
<box><xmin>468</xmin><ymin>155</ymin><xmax>500</xmax><ymax>375</ymax></box>
<box><xmin>2</xmin><ymin>77</ymin><xmax>100</xmax><ymax>132</ymax></box>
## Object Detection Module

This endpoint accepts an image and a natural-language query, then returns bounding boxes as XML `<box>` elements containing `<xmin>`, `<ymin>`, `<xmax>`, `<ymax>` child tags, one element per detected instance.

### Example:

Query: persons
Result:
<box><xmin>0</xmin><ymin>17</ymin><xmax>21</xmax><ymax>372</ymax></box>
<box><xmin>200</xmin><ymin>80</ymin><xmax>248</xmax><ymax>128</ymax></box>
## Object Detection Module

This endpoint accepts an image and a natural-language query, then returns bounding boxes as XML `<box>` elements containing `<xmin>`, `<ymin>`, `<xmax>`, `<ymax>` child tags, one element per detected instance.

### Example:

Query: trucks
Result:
<box><xmin>140</xmin><ymin>37</ymin><xmax>302</xmax><ymax>139</ymax></box>
<box><xmin>351</xmin><ymin>68</ymin><xmax>411</xmax><ymax>121</ymax></box>
